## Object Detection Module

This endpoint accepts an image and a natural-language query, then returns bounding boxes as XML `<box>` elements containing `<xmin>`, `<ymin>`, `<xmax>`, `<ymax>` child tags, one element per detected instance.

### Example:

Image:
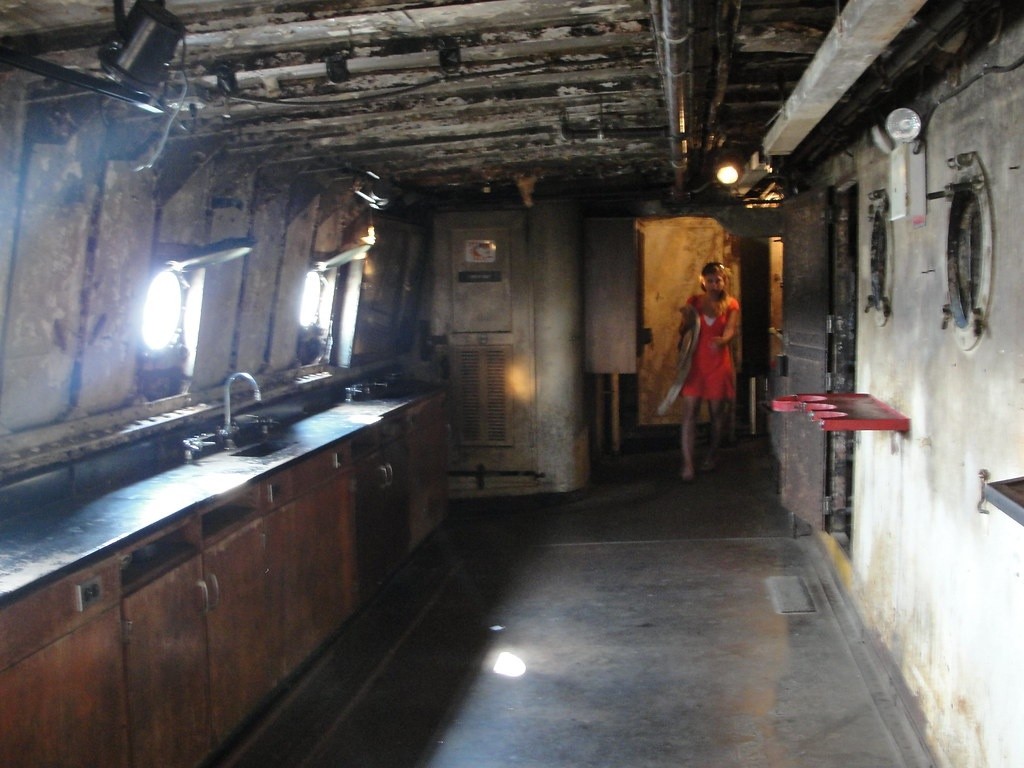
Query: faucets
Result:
<box><xmin>219</xmin><ymin>372</ymin><xmax>262</xmax><ymax>452</ymax></box>
<box><xmin>364</xmin><ymin>381</ymin><xmax>388</xmax><ymax>388</ymax></box>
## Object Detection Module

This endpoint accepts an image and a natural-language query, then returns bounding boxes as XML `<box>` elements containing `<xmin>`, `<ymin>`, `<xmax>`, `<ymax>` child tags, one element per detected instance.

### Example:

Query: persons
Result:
<box><xmin>679</xmin><ymin>262</ymin><xmax>739</xmax><ymax>481</ymax></box>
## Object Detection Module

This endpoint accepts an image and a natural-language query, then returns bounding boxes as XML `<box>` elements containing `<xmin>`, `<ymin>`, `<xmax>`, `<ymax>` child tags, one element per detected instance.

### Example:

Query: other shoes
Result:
<box><xmin>683</xmin><ymin>465</ymin><xmax>694</xmax><ymax>483</ymax></box>
<box><xmin>701</xmin><ymin>452</ymin><xmax>718</xmax><ymax>471</ymax></box>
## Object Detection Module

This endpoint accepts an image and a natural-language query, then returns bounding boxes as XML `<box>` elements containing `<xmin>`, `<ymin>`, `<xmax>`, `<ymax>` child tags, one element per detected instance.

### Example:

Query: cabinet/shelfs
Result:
<box><xmin>1</xmin><ymin>389</ymin><xmax>454</xmax><ymax>766</ymax></box>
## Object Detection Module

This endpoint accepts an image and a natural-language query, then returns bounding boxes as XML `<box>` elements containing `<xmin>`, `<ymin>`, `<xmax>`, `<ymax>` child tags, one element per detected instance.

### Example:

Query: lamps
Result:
<box><xmin>98</xmin><ymin>0</ymin><xmax>187</xmax><ymax>97</ymax></box>
<box><xmin>869</xmin><ymin>105</ymin><xmax>925</xmax><ymax>157</ymax></box>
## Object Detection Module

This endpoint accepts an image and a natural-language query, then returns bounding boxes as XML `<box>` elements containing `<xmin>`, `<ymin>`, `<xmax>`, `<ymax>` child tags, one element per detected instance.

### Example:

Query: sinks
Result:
<box><xmin>229</xmin><ymin>440</ymin><xmax>301</xmax><ymax>458</ymax></box>
